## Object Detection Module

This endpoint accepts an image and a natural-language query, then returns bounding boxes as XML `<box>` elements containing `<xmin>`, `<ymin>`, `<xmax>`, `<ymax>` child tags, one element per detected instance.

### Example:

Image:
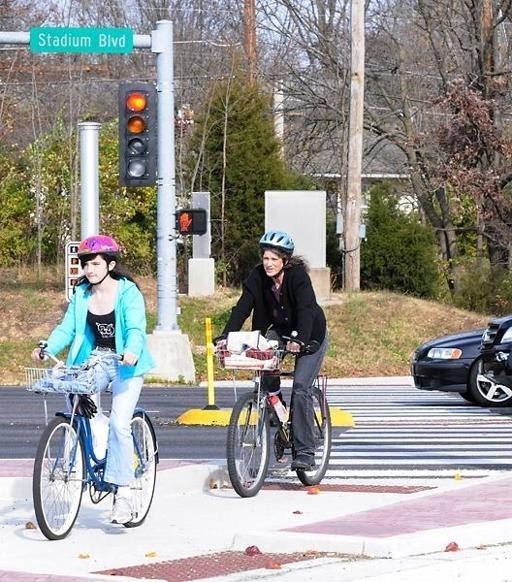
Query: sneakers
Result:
<box><xmin>270</xmin><ymin>401</ymin><xmax>287</xmax><ymax>428</ymax></box>
<box><xmin>109</xmin><ymin>494</ymin><xmax>135</xmax><ymax>525</ymax></box>
<box><xmin>291</xmin><ymin>454</ymin><xmax>316</xmax><ymax>469</ymax></box>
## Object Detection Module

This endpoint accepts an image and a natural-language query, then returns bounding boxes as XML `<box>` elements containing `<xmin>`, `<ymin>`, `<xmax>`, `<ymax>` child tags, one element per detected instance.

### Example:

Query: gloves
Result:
<box><xmin>69</xmin><ymin>393</ymin><xmax>97</xmax><ymax>418</ymax></box>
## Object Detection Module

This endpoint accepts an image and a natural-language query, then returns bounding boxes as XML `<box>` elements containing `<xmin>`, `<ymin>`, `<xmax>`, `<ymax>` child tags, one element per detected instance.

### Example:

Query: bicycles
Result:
<box><xmin>23</xmin><ymin>340</ymin><xmax>159</xmax><ymax>540</ymax></box>
<box><xmin>218</xmin><ymin>330</ymin><xmax>331</xmax><ymax>498</ymax></box>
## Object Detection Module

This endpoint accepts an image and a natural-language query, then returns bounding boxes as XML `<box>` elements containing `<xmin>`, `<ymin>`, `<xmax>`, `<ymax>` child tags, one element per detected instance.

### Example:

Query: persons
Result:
<box><xmin>212</xmin><ymin>230</ymin><xmax>329</xmax><ymax>471</ymax></box>
<box><xmin>29</xmin><ymin>234</ymin><xmax>159</xmax><ymax>523</ymax></box>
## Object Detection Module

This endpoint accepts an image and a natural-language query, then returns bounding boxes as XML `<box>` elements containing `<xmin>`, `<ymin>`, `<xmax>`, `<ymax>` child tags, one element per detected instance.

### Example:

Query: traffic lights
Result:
<box><xmin>118</xmin><ymin>82</ymin><xmax>157</xmax><ymax>187</ymax></box>
<box><xmin>176</xmin><ymin>209</ymin><xmax>208</xmax><ymax>235</ymax></box>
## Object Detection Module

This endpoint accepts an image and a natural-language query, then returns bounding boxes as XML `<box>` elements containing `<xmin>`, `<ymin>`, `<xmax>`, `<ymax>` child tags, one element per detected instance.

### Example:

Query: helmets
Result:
<box><xmin>259</xmin><ymin>230</ymin><xmax>295</xmax><ymax>254</ymax></box>
<box><xmin>77</xmin><ymin>235</ymin><xmax>118</xmax><ymax>258</ymax></box>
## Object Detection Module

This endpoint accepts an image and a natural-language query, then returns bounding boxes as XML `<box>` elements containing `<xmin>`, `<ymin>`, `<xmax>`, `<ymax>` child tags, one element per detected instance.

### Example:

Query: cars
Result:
<box><xmin>410</xmin><ymin>314</ymin><xmax>512</xmax><ymax>408</ymax></box>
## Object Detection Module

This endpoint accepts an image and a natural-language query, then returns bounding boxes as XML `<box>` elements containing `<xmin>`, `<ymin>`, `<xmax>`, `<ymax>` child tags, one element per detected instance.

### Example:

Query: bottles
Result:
<box><xmin>270</xmin><ymin>395</ymin><xmax>289</xmax><ymax>423</ymax></box>
